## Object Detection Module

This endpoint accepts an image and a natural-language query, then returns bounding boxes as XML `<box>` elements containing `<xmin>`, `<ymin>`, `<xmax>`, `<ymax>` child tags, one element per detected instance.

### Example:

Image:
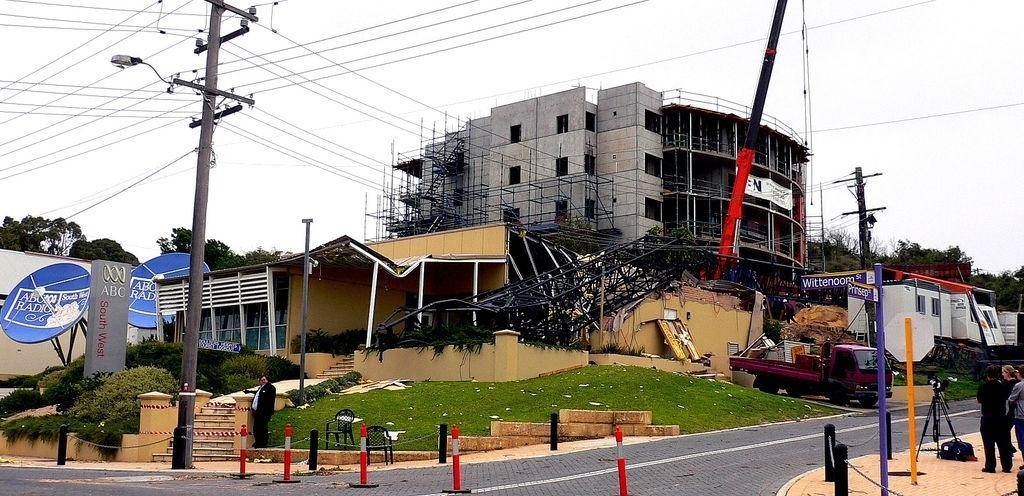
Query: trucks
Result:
<box><xmin>729</xmin><ymin>342</ymin><xmax>896</xmax><ymax>410</ymax></box>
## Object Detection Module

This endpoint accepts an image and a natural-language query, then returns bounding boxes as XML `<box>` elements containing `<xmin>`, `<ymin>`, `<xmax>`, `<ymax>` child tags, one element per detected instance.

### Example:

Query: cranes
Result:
<box><xmin>693</xmin><ymin>0</ymin><xmax>826</xmax><ymax>295</ymax></box>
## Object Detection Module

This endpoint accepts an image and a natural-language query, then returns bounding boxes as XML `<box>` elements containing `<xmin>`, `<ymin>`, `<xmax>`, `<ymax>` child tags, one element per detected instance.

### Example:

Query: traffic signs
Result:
<box><xmin>795</xmin><ymin>270</ymin><xmax>878</xmax><ymax>292</ymax></box>
<box><xmin>841</xmin><ymin>279</ymin><xmax>884</xmax><ymax>303</ymax></box>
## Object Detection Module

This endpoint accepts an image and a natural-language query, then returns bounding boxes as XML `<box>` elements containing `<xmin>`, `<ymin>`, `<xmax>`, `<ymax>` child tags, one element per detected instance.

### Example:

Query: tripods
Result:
<box><xmin>916</xmin><ymin>396</ymin><xmax>959</xmax><ymax>460</ymax></box>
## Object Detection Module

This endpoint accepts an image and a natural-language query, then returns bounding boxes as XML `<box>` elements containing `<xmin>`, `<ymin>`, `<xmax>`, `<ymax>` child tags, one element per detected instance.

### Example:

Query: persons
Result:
<box><xmin>783</xmin><ymin>303</ymin><xmax>796</xmax><ymax>325</ymax></box>
<box><xmin>978</xmin><ymin>363</ymin><xmax>1023</xmax><ymax>473</ymax></box>
<box><xmin>251</xmin><ymin>376</ymin><xmax>276</xmax><ymax>448</ymax></box>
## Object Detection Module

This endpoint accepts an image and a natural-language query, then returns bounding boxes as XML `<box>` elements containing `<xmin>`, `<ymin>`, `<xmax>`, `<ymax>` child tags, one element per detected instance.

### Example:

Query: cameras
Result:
<box><xmin>925</xmin><ymin>376</ymin><xmax>948</xmax><ymax>393</ymax></box>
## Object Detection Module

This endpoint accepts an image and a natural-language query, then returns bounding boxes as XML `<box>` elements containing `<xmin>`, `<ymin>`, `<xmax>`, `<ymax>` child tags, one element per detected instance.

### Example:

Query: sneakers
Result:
<box><xmin>982</xmin><ymin>466</ymin><xmax>996</xmax><ymax>472</ymax></box>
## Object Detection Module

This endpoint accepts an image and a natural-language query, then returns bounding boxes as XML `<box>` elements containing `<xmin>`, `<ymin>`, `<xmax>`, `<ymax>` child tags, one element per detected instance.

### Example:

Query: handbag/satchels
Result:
<box><xmin>940</xmin><ymin>437</ymin><xmax>975</xmax><ymax>461</ymax></box>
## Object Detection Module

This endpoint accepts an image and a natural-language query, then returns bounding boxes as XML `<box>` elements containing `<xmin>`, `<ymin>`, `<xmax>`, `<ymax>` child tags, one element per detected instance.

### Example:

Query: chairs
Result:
<box><xmin>363</xmin><ymin>424</ymin><xmax>396</xmax><ymax>467</ymax></box>
<box><xmin>324</xmin><ymin>409</ymin><xmax>359</xmax><ymax>451</ymax></box>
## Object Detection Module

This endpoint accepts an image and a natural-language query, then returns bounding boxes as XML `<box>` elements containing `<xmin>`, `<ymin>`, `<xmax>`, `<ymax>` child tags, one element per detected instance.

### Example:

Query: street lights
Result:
<box><xmin>102</xmin><ymin>0</ymin><xmax>227</xmax><ymax>477</ymax></box>
<box><xmin>296</xmin><ymin>216</ymin><xmax>319</xmax><ymax>408</ymax></box>
